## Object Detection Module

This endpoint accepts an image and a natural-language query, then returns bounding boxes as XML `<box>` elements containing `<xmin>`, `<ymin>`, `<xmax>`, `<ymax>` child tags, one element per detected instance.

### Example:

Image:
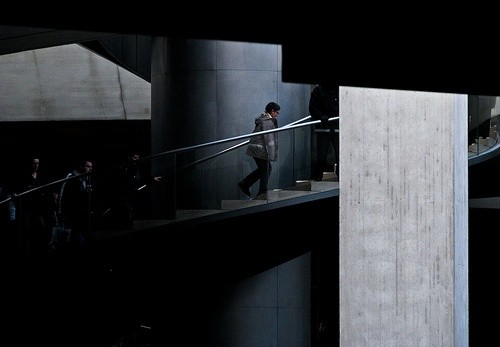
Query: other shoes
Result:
<box><xmin>238</xmin><ymin>182</ymin><xmax>251</xmax><ymax>195</ymax></box>
<box><xmin>315</xmin><ymin>176</ymin><xmax>323</xmax><ymax>181</ymax></box>
<box><xmin>257</xmin><ymin>193</ymin><xmax>271</xmax><ymax>199</ymax></box>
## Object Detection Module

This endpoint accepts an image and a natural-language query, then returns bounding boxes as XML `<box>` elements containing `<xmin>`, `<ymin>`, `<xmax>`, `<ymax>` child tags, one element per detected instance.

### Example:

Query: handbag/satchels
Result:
<box><xmin>51</xmin><ymin>212</ymin><xmax>72</xmax><ymax>244</ymax></box>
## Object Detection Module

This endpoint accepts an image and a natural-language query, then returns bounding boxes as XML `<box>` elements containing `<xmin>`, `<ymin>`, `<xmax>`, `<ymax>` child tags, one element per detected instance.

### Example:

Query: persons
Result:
<box><xmin>8</xmin><ymin>136</ymin><xmax>166</xmax><ymax>261</ymax></box>
<box><xmin>235</xmin><ymin>101</ymin><xmax>283</xmax><ymax>203</ymax></box>
<box><xmin>307</xmin><ymin>72</ymin><xmax>338</xmax><ymax>182</ymax></box>
